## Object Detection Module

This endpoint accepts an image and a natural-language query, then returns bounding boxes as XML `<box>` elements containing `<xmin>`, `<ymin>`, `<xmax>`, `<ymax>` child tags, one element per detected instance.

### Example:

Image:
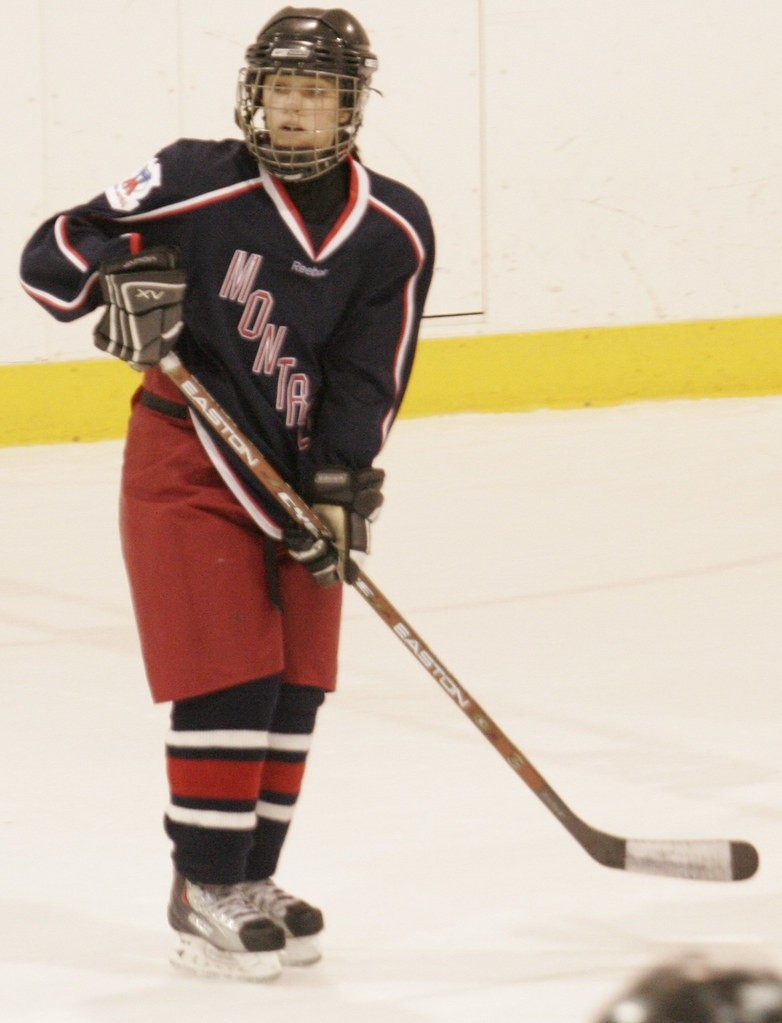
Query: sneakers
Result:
<box><xmin>236</xmin><ymin>879</ymin><xmax>325</xmax><ymax>968</ymax></box>
<box><xmin>166</xmin><ymin>870</ymin><xmax>286</xmax><ymax>980</ymax></box>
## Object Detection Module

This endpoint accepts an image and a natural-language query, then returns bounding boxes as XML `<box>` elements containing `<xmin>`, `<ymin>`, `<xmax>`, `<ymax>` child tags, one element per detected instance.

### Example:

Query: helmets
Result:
<box><xmin>234</xmin><ymin>3</ymin><xmax>377</xmax><ymax>182</ymax></box>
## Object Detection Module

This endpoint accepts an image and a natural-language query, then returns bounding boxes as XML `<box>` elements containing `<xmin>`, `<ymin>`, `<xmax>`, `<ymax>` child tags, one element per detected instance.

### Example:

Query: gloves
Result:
<box><xmin>93</xmin><ymin>246</ymin><xmax>188</xmax><ymax>368</ymax></box>
<box><xmin>277</xmin><ymin>465</ymin><xmax>382</xmax><ymax>585</ymax></box>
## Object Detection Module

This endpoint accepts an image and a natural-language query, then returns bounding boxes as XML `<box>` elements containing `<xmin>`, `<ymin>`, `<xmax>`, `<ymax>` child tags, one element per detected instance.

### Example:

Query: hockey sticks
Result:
<box><xmin>159</xmin><ymin>352</ymin><xmax>761</xmax><ymax>885</ymax></box>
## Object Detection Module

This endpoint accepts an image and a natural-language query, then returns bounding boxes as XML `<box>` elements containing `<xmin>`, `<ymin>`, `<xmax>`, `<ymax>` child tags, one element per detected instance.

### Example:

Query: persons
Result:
<box><xmin>21</xmin><ymin>9</ymin><xmax>435</xmax><ymax>984</ymax></box>
